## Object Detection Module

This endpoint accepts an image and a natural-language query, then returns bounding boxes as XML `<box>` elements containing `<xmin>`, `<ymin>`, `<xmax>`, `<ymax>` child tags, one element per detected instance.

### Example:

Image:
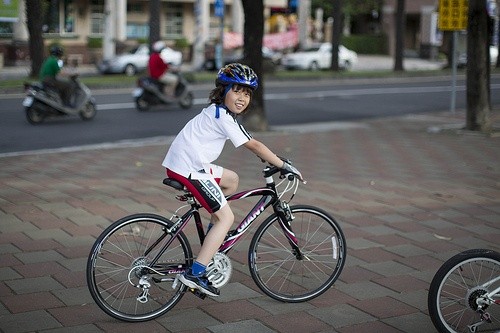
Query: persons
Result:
<box><xmin>149</xmin><ymin>40</ymin><xmax>177</xmax><ymax>96</ymax></box>
<box><xmin>161</xmin><ymin>62</ymin><xmax>302</xmax><ymax>297</ymax></box>
<box><xmin>38</xmin><ymin>45</ymin><xmax>72</xmax><ymax>106</ymax></box>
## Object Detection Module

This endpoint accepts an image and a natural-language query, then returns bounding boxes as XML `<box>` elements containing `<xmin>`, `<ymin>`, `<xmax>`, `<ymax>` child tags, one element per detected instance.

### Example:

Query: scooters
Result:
<box><xmin>21</xmin><ymin>59</ymin><xmax>98</xmax><ymax>126</ymax></box>
<box><xmin>131</xmin><ymin>62</ymin><xmax>195</xmax><ymax>113</ymax></box>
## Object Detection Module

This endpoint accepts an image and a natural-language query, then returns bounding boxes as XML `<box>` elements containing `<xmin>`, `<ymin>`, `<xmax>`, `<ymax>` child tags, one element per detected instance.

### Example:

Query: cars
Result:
<box><xmin>456</xmin><ymin>43</ymin><xmax>499</xmax><ymax>68</ymax></box>
<box><xmin>203</xmin><ymin>43</ymin><xmax>282</xmax><ymax>75</ymax></box>
<box><xmin>278</xmin><ymin>39</ymin><xmax>359</xmax><ymax>73</ymax></box>
<box><xmin>96</xmin><ymin>43</ymin><xmax>184</xmax><ymax>78</ymax></box>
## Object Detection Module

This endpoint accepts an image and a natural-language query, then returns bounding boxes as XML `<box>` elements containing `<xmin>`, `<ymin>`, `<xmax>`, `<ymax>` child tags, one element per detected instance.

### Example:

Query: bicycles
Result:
<box><xmin>427</xmin><ymin>248</ymin><xmax>500</xmax><ymax>333</ymax></box>
<box><xmin>86</xmin><ymin>155</ymin><xmax>347</xmax><ymax>323</ymax></box>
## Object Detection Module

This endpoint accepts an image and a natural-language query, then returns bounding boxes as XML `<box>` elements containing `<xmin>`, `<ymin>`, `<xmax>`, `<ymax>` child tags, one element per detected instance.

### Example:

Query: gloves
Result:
<box><xmin>280</xmin><ymin>161</ymin><xmax>304</xmax><ymax>184</ymax></box>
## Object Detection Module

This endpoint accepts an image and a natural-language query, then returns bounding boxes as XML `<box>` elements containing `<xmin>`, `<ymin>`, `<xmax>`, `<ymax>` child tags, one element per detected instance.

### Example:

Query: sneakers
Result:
<box><xmin>205</xmin><ymin>228</ymin><xmax>236</xmax><ymax>241</ymax></box>
<box><xmin>178</xmin><ymin>265</ymin><xmax>221</xmax><ymax>297</ymax></box>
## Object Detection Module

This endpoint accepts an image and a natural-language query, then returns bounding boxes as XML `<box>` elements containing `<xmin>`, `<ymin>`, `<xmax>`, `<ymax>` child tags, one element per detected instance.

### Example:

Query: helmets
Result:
<box><xmin>152</xmin><ymin>41</ymin><xmax>166</xmax><ymax>52</ymax></box>
<box><xmin>49</xmin><ymin>43</ymin><xmax>65</xmax><ymax>56</ymax></box>
<box><xmin>215</xmin><ymin>63</ymin><xmax>260</xmax><ymax>90</ymax></box>
